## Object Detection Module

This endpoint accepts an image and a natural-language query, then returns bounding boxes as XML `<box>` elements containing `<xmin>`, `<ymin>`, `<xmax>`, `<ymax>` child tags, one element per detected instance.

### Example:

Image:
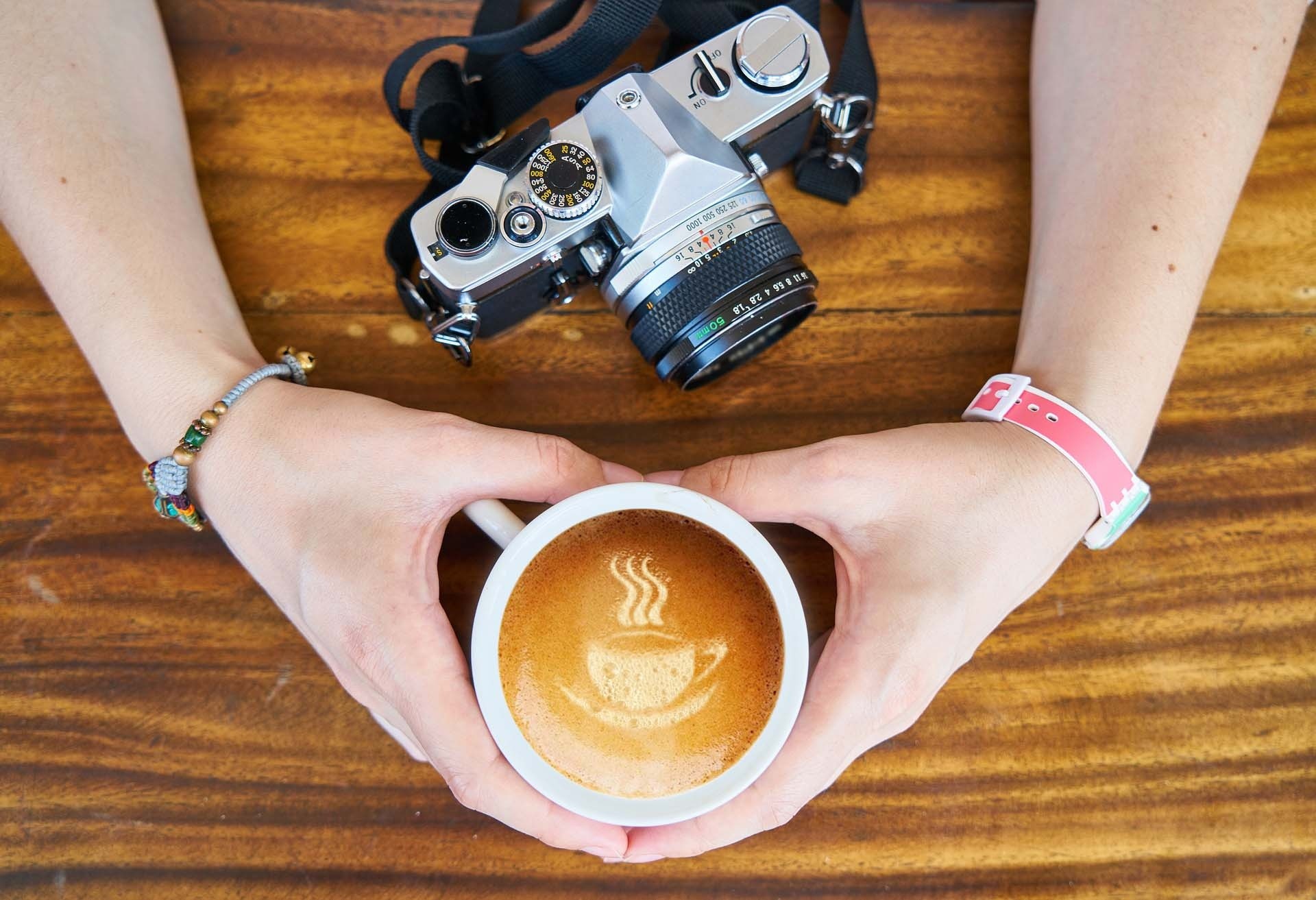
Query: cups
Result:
<box><xmin>471</xmin><ymin>480</ymin><xmax>810</xmax><ymax>829</ymax></box>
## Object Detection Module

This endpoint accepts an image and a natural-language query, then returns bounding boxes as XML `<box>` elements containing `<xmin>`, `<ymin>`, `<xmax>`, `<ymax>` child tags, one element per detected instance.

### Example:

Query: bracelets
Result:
<box><xmin>144</xmin><ymin>347</ymin><xmax>313</xmax><ymax>531</ymax></box>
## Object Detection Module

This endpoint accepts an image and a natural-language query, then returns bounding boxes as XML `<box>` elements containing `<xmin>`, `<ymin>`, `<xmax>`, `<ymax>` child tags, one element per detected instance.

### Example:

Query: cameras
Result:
<box><xmin>384</xmin><ymin>4</ymin><xmax>831</xmax><ymax>391</ymax></box>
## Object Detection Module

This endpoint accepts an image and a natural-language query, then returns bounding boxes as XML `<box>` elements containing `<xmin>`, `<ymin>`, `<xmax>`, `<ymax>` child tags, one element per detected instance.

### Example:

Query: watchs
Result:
<box><xmin>961</xmin><ymin>374</ymin><xmax>1154</xmax><ymax>553</ymax></box>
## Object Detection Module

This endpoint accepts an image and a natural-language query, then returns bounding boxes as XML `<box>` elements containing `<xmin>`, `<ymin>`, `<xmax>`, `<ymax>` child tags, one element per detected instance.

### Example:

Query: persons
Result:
<box><xmin>0</xmin><ymin>0</ymin><xmax>1316</xmax><ymax>863</ymax></box>
